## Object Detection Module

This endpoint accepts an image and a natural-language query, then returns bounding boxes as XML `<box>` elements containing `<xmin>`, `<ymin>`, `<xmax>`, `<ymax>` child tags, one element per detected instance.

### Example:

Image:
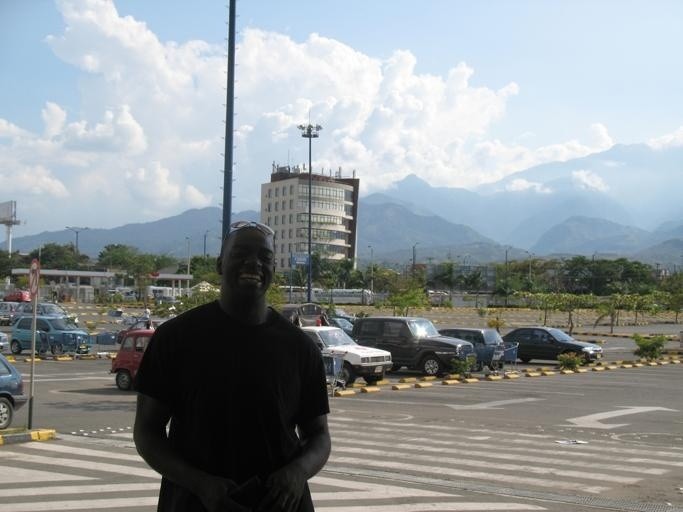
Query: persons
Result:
<box><xmin>132</xmin><ymin>218</ymin><xmax>332</xmax><ymax>512</ymax></box>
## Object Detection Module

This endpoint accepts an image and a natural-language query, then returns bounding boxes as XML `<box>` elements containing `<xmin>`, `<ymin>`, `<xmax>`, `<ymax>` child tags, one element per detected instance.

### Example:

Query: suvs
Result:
<box><xmin>0</xmin><ymin>289</ymin><xmax>90</xmax><ymax>358</ymax></box>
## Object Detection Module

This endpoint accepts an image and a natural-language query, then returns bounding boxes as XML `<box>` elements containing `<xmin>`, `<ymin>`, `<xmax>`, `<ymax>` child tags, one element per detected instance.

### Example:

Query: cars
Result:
<box><xmin>109</xmin><ymin>330</ymin><xmax>154</xmax><ymax>390</ymax></box>
<box><xmin>0</xmin><ymin>350</ymin><xmax>28</xmax><ymax>432</ymax></box>
<box><xmin>88</xmin><ymin>319</ymin><xmax>164</xmax><ymax>354</ymax></box>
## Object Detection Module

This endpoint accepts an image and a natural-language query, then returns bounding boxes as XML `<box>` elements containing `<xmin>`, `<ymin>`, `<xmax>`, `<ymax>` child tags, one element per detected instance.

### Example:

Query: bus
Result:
<box><xmin>382</xmin><ymin>288</ymin><xmax>449</xmax><ymax>306</ymax></box>
<box><xmin>278</xmin><ymin>285</ymin><xmax>374</xmax><ymax>306</ymax></box>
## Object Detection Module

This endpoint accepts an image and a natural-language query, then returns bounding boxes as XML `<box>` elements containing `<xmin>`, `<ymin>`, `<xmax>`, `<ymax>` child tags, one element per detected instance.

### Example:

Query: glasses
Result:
<box><xmin>223</xmin><ymin>221</ymin><xmax>278</xmax><ymax>244</ymax></box>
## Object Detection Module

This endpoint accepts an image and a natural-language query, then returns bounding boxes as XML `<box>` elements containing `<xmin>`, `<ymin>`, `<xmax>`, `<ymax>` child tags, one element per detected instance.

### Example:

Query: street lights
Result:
<box><xmin>294</xmin><ymin>110</ymin><xmax>323</xmax><ymax>302</ymax></box>
<box><xmin>366</xmin><ymin>245</ymin><xmax>374</xmax><ymax>292</ymax></box>
<box><xmin>202</xmin><ymin>228</ymin><xmax>211</xmax><ymax>258</ymax></box>
<box><xmin>411</xmin><ymin>241</ymin><xmax>421</xmax><ymax>270</ymax></box>
<box><xmin>524</xmin><ymin>250</ymin><xmax>536</xmax><ymax>282</ymax></box>
<box><xmin>64</xmin><ymin>226</ymin><xmax>89</xmax><ymax>261</ymax></box>
<box><xmin>654</xmin><ymin>262</ymin><xmax>660</xmax><ymax>274</ymax></box>
<box><xmin>185</xmin><ymin>235</ymin><xmax>192</xmax><ymax>289</ymax></box>
